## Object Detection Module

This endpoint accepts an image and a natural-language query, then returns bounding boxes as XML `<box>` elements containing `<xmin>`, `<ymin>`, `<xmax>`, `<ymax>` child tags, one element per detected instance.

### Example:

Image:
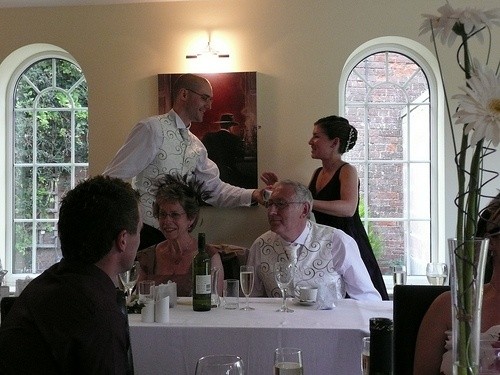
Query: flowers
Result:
<box><xmin>418</xmin><ymin>2</ymin><xmax>499</xmax><ymax>374</ymax></box>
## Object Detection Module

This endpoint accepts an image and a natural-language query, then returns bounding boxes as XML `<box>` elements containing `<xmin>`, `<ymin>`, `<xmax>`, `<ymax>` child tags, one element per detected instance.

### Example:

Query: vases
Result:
<box><xmin>450</xmin><ymin>237</ymin><xmax>489</xmax><ymax>374</ymax></box>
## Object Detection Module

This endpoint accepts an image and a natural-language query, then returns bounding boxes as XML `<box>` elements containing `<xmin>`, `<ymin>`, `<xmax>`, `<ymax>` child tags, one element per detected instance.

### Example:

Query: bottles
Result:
<box><xmin>192</xmin><ymin>233</ymin><xmax>212</xmax><ymax>311</ymax></box>
<box><xmin>368</xmin><ymin>318</ymin><xmax>395</xmax><ymax>375</ymax></box>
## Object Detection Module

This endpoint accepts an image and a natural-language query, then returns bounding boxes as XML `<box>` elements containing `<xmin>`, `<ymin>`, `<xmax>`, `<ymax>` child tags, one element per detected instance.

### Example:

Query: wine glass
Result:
<box><xmin>240</xmin><ymin>266</ymin><xmax>256</xmax><ymax>311</ymax></box>
<box><xmin>274</xmin><ymin>262</ymin><xmax>294</xmax><ymax>312</ymax></box>
<box><xmin>118</xmin><ymin>260</ymin><xmax>141</xmax><ymax>304</ymax></box>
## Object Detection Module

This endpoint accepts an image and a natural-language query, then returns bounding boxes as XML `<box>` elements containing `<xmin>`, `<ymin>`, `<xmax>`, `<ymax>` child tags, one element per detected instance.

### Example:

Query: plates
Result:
<box><xmin>293</xmin><ymin>299</ymin><xmax>320</xmax><ymax>306</ymax></box>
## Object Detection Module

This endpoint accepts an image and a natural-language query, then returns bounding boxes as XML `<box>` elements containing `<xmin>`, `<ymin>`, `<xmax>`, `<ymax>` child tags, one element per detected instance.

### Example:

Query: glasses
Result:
<box><xmin>158</xmin><ymin>210</ymin><xmax>187</xmax><ymax>217</ymax></box>
<box><xmin>268</xmin><ymin>200</ymin><xmax>305</xmax><ymax>208</ymax></box>
<box><xmin>186</xmin><ymin>89</ymin><xmax>214</xmax><ymax>103</ymax></box>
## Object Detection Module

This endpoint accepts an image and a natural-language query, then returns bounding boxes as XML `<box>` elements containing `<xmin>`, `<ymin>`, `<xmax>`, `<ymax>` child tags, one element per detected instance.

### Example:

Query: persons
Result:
<box><xmin>137</xmin><ymin>182</ymin><xmax>224</xmax><ymax>296</ymax></box>
<box><xmin>102</xmin><ymin>74</ymin><xmax>264</xmax><ymax>294</ymax></box>
<box><xmin>260</xmin><ymin>115</ymin><xmax>389</xmax><ymax>300</ymax></box>
<box><xmin>0</xmin><ymin>176</ymin><xmax>143</xmax><ymax>375</ymax></box>
<box><xmin>414</xmin><ymin>194</ymin><xmax>500</xmax><ymax>375</ymax></box>
<box><xmin>245</xmin><ymin>180</ymin><xmax>381</xmax><ymax>300</ymax></box>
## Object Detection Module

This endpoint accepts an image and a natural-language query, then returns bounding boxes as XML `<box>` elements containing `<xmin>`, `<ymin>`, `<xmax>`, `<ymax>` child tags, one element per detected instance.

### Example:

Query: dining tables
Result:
<box><xmin>128</xmin><ymin>295</ymin><xmax>393</xmax><ymax>375</ymax></box>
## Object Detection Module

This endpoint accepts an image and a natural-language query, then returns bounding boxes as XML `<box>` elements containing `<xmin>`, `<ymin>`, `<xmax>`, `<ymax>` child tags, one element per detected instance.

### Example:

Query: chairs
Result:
<box><xmin>393</xmin><ymin>286</ymin><xmax>449</xmax><ymax>375</ymax></box>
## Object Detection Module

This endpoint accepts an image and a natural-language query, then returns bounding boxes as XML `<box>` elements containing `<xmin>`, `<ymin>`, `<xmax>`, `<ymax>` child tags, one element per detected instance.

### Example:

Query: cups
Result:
<box><xmin>223</xmin><ymin>280</ymin><xmax>239</xmax><ymax>310</ymax></box>
<box><xmin>263</xmin><ymin>183</ymin><xmax>273</xmax><ymax>199</ymax></box>
<box><xmin>138</xmin><ymin>280</ymin><xmax>177</xmax><ymax>324</ymax></box>
<box><xmin>426</xmin><ymin>263</ymin><xmax>449</xmax><ymax>286</ymax></box>
<box><xmin>295</xmin><ymin>285</ymin><xmax>319</xmax><ymax>303</ymax></box>
<box><xmin>208</xmin><ymin>266</ymin><xmax>221</xmax><ymax>308</ymax></box>
<box><xmin>274</xmin><ymin>347</ymin><xmax>304</xmax><ymax>375</ymax></box>
<box><xmin>392</xmin><ymin>265</ymin><xmax>407</xmax><ymax>285</ymax></box>
<box><xmin>361</xmin><ymin>337</ymin><xmax>369</xmax><ymax>375</ymax></box>
<box><xmin>195</xmin><ymin>354</ymin><xmax>247</xmax><ymax>375</ymax></box>
<box><xmin>468</xmin><ymin>332</ymin><xmax>500</xmax><ymax>375</ymax></box>
